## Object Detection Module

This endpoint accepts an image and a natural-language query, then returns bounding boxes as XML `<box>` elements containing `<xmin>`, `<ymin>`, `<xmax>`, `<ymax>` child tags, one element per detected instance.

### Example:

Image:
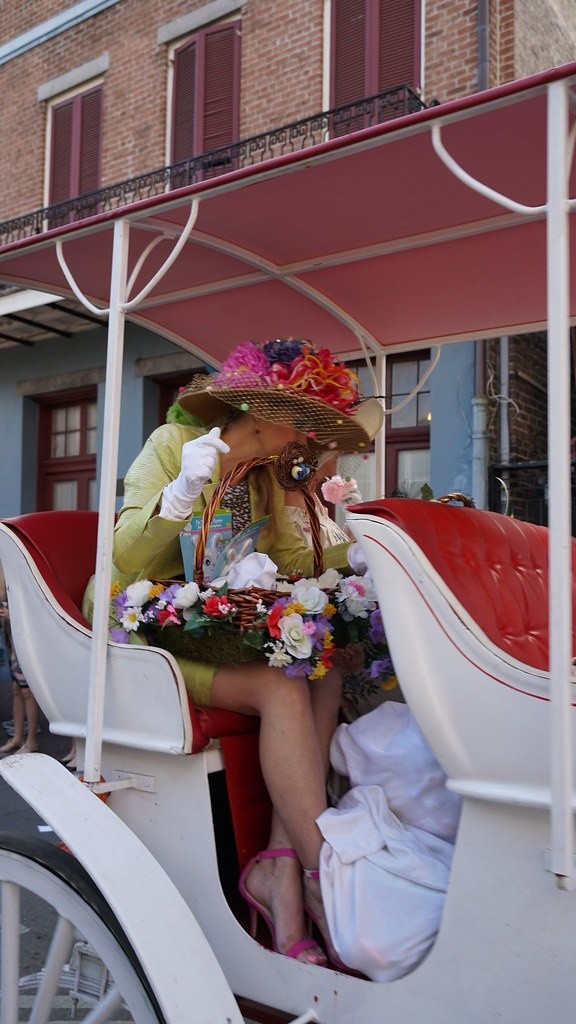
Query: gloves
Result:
<box><xmin>347</xmin><ymin>541</ymin><xmax>368</xmax><ymax>576</ymax></box>
<box><xmin>159</xmin><ymin>426</ymin><xmax>230</xmax><ymax>521</ymax></box>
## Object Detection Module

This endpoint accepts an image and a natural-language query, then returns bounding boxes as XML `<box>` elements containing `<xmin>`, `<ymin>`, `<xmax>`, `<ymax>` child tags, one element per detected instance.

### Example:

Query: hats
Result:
<box><xmin>177</xmin><ymin>334</ymin><xmax>384</xmax><ymax>453</ymax></box>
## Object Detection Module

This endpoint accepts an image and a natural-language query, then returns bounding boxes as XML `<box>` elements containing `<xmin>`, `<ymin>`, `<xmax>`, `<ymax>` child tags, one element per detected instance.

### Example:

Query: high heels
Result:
<box><xmin>301</xmin><ymin>869</ymin><xmax>367</xmax><ymax>979</ymax></box>
<box><xmin>238</xmin><ymin>848</ymin><xmax>328</xmax><ymax>969</ymax></box>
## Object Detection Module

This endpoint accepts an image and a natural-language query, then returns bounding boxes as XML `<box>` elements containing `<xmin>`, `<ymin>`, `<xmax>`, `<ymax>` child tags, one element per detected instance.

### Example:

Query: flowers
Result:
<box><xmin>108</xmin><ymin>475</ymin><xmax>392</xmax><ymax>682</ymax></box>
<box><xmin>217</xmin><ymin>335</ymin><xmax>365</xmax><ymax>418</ymax></box>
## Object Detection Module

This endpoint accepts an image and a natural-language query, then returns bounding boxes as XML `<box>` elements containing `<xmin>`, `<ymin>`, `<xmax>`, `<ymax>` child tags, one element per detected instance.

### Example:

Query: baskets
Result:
<box><xmin>150</xmin><ymin>439</ymin><xmax>340</xmax><ymax>662</ymax></box>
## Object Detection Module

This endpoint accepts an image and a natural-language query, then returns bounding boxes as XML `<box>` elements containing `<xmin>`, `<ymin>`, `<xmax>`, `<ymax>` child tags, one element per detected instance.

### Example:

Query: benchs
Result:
<box><xmin>0</xmin><ymin>498</ymin><xmax>576</xmax><ymax>806</ymax></box>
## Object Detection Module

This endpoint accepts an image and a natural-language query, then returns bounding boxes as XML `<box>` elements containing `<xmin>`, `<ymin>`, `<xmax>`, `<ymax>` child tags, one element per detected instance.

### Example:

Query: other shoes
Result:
<box><xmin>64</xmin><ymin>761</ymin><xmax>77</xmax><ymax>771</ymax></box>
<box><xmin>57</xmin><ymin>757</ymin><xmax>71</xmax><ymax>765</ymax></box>
<box><xmin>0</xmin><ymin>738</ymin><xmax>24</xmax><ymax>752</ymax></box>
<box><xmin>6</xmin><ymin>724</ymin><xmax>40</xmax><ymax>736</ymax></box>
<box><xmin>2</xmin><ymin>719</ymin><xmax>28</xmax><ymax>728</ymax></box>
<box><xmin>13</xmin><ymin>743</ymin><xmax>38</xmax><ymax>755</ymax></box>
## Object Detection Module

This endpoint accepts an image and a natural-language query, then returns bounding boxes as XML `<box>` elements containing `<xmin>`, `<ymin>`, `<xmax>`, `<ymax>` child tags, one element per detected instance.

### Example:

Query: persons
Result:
<box><xmin>84</xmin><ymin>395</ymin><xmax>367</xmax><ymax>969</ymax></box>
<box><xmin>0</xmin><ymin>561</ymin><xmax>40</xmax><ymax>755</ymax></box>
<box><xmin>58</xmin><ymin>736</ymin><xmax>77</xmax><ymax>773</ymax></box>
